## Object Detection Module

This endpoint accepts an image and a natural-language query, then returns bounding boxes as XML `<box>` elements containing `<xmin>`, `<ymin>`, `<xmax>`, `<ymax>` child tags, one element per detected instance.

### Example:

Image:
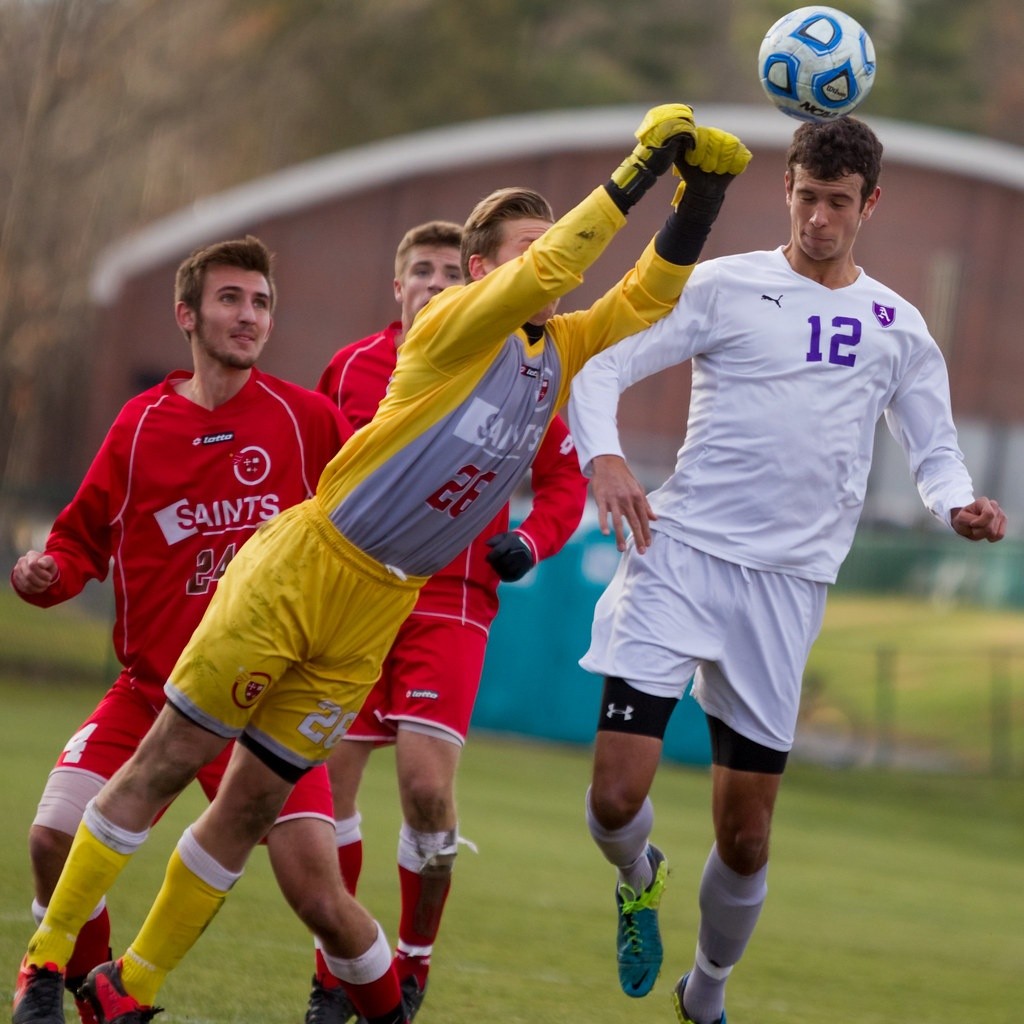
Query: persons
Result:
<box><xmin>568</xmin><ymin>117</ymin><xmax>1007</xmax><ymax>1024</ymax></box>
<box><xmin>13</xmin><ymin>104</ymin><xmax>752</xmax><ymax>1024</ymax></box>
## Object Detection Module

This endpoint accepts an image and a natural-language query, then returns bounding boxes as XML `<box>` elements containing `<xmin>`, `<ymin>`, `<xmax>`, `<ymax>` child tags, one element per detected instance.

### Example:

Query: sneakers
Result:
<box><xmin>77</xmin><ymin>959</ymin><xmax>164</xmax><ymax>1024</ymax></box>
<box><xmin>354</xmin><ymin>974</ymin><xmax>428</xmax><ymax>1024</ymax></box>
<box><xmin>305</xmin><ymin>973</ymin><xmax>369</xmax><ymax>1024</ymax></box>
<box><xmin>615</xmin><ymin>843</ymin><xmax>670</xmax><ymax>998</ymax></box>
<box><xmin>10</xmin><ymin>953</ymin><xmax>67</xmax><ymax>1024</ymax></box>
<box><xmin>670</xmin><ymin>972</ymin><xmax>726</xmax><ymax>1024</ymax></box>
<box><xmin>65</xmin><ymin>947</ymin><xmax>113</xmax><ymax>1024</ymax></box>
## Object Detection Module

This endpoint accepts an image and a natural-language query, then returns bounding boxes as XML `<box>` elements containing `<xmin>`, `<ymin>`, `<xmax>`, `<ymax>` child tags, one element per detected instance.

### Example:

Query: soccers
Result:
<box><xmin>758</xmin><ymin>5</ymin><xmax>876</xmax><ymax>124</ymax></box>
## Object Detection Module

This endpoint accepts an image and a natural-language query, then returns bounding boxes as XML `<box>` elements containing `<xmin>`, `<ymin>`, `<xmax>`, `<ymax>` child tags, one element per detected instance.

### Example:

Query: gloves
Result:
<box><xmin>604</xmin><ymin>103</ymin><xmax>697</xmax><ymax>216</ymax></box>
<box><xmin>483</xmin><ymin>533</ymin><xmax>533</xmax><ymax>582</ymax></box>
<box><xmin>654</xmin><ymin>124</ymin><xmax>753</xmax><ymax>265</ymax></box>
